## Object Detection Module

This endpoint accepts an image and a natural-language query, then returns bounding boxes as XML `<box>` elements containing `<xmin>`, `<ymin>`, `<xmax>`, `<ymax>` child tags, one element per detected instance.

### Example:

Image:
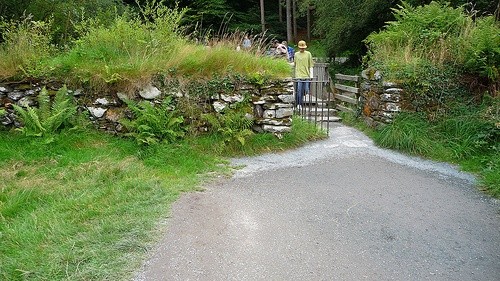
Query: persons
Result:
<box><xmin>280</xmin><ymin>41</ymin><xmax>294</xmax><ymax>62</ymax></box>
<box><xmin>242</xmin><ymin>35</ymin><xmax>250</xmax><ymax>47</ymax></box>
<box><xmin>293</xmin><ymin>40</ymin><xmax>314</xmax><ymax>110</ymax></box>
<box><xmin>272</xmin><ymin>39</ymin><xmax>288</xmax><ymax>61</ymax></box>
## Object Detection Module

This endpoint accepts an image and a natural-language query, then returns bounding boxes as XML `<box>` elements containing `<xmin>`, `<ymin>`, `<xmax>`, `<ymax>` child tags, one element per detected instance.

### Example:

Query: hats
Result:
<box><xmin>298</xmin><ymin>41</ymin><xmax>307</xmax><ymax>48</ymax></box>
